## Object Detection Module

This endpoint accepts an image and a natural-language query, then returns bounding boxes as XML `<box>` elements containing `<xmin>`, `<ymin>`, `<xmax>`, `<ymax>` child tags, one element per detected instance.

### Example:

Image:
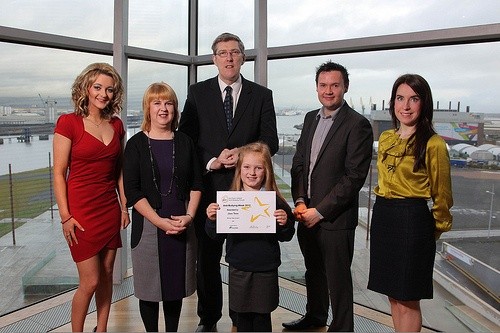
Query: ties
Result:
<box><xmin>223</xmin><ymin>86</ymin><xmax>234</xmax><ymax>134</ymax></box>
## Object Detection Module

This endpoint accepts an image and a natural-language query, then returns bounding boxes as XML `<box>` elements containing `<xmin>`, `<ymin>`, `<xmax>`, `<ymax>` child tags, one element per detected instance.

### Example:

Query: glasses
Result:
<box><xmin>216</xmin><ymin>50</ymin><xmax>243</xmax><ymax>57</ymax></box>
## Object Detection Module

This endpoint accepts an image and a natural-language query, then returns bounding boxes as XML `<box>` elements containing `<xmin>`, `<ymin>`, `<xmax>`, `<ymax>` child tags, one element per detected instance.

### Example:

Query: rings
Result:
<box><xmin>67</xmin><ymin>233</ymin><xmax>71</xmax><ymax>237</ymax></box>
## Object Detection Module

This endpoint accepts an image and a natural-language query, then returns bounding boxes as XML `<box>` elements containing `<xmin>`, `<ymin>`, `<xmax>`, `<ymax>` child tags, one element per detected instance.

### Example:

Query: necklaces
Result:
<box><xmin>148</xmin><ymin>130</ymin><xmax>175</xmax><ymax>196</ymax></box>
<box><xmin>87</xmin><ymin>117</ymin><xmax>103</xmax><ymax>127</ymax></box>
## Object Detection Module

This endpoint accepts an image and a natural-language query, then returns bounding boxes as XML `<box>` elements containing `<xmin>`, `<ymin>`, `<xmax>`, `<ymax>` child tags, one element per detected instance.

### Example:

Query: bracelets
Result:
<box><xmin>186</xmin><ymin>214</ymin><xmax>194</xmax><ymax>226</ymax></box>
<box><xmin>60</xmin><ymin>215</ymin><xmax>73</xmax><ymax>224</ymax></box>
<box><xmin>121</xmin><ymin>210</ymin><xmax>129</xmax><ymax>215</ymax></box>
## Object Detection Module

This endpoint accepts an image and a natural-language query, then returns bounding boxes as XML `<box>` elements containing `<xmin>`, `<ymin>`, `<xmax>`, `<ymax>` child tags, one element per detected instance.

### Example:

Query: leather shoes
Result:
<box><xmin>192</xmin><ymin>322</ymin><xmax>217</xmax><ymax>333</ymax></box>
<box><xmin>281</xmin><ymin>315</ymin><xmax>327</xmax><ymax>331</ymax></box>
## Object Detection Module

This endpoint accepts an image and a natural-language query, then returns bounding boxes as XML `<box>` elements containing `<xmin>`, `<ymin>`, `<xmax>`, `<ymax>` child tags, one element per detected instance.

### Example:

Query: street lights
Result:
<box><xmin>485</xmin><ymin>184</ymin><xmax>495</xmax><ymax>239</ymax></box>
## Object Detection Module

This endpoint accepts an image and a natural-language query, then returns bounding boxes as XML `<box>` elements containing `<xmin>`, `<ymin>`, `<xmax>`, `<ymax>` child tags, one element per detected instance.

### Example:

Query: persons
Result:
<box><xmin>282</xmin><ymin>61</ymin><xmax>374</xmax><ymax>333</ymax></box>
<box><xmin>53</xmin><ymin>63</ymin><xmax>130</xmax><ymax>333</ymax></box>
<box><xmin>178</xmin><ymin>33</ymin><xmax>279</xmax><ymax>333</ymax></box>
<box><xmin>205</xmin><ymin>142</ymin><xmax>295</xmax><ymax>333</ymax></box>
<box><xmin>367</xmin><ymin>74</ymin><xmax>454</xmax><ymax>333</ymax></box>
<box><xmin>123</xmin><ymin>82</ymin><xmax>204</xmax><ymax>333</ymax></box>
